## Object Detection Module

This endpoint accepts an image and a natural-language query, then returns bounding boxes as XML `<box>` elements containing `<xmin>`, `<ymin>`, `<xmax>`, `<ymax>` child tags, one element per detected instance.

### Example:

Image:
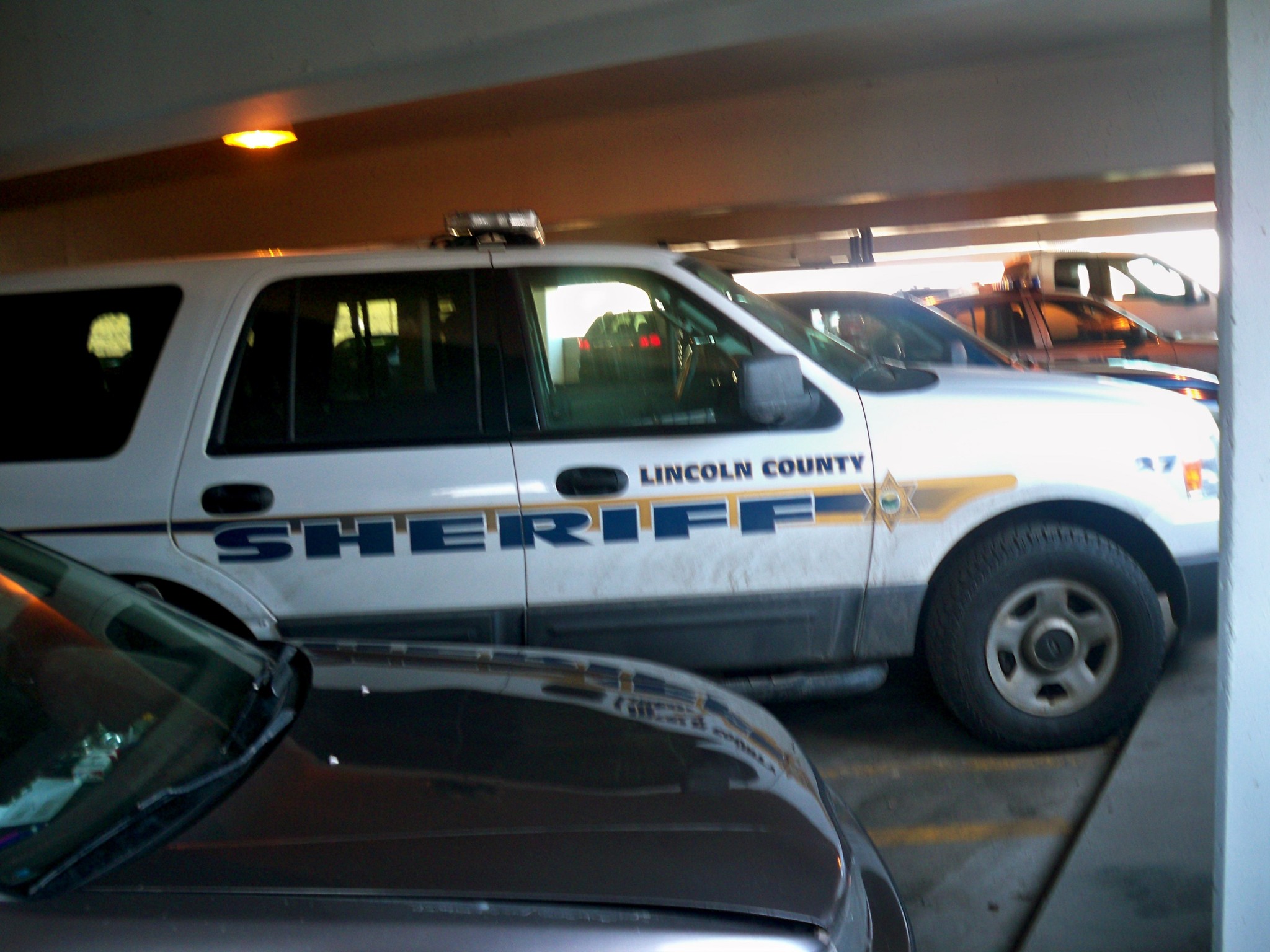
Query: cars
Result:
<box><xmin>1</xmin><ymin>526</ymin><xmax>917</xmax><ymax>951</ymax></box>
<box><xmin>580</xmin><ymin>257</ymin><xmax>1221</xmax><ymax>387</ymax></box>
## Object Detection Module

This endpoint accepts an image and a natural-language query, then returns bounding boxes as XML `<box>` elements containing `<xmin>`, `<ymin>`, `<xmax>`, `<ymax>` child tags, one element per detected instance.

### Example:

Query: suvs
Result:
<box><xmin>0</xmin><ymin>207</ymin><xmax>1222</xmax><ymax>759</ymax></box>
